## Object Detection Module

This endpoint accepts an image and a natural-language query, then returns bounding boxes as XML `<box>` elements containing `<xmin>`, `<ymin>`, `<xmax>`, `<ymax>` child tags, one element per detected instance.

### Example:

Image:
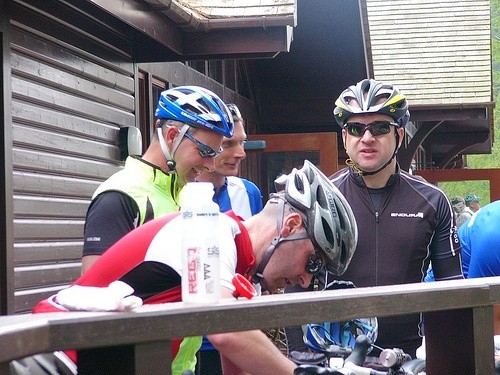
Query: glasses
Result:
<box><xmin>166</xmin><ymin>124</ymin><xmax>224</xmax><ymax>159</ymax></box>
<box><xmin>290</xmin><ymin>206</ymin><xmax>324</xmax><ymax>275</ymax></box>
<box><xmin>342</xmin><ymin>120</ymin><xmax>400</xmax><ymax>137</ymax></box>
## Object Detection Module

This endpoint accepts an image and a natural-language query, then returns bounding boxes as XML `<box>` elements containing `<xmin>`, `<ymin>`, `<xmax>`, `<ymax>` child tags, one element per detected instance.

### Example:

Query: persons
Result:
<box><xmin>423</xmin><ymin>193</ymin><xmax>500</xmax><ymax>375</ymax></box>
<box><xmin>284</xmin><ymin>78</ymin><xmax>465</xmax><ymax>360</ymax></box>
<box><xmin>9</xmin><ymin>159</ymin><xmax>358</xmax><ymax>375</ymax></box>
<box><xmin>80</xmin><ymin>85</ymin><xmax>234</xmax><ymax>278</ymax></box>
<box><xmin>194</xmin><ymin>103</ymin><xmax>278</xmax><ymax>375</ymax></box>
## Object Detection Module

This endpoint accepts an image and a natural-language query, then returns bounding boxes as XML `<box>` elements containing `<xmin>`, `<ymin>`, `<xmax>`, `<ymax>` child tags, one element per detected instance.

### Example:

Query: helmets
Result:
<box><xmin>333</xmin><ymin>78</ymin><xmax>411</xmax><ymax>128</ymax></box>
<box><xmin>154</xmin><ymin>85</ymin><xmax>235</xmax><ymax>138</ymax></box>
<box><xmin>273</xmin><ymin>159</ymin><xmax>358</xmax><ymax>277</ymax></box>
<box><xmin>451</xmin><ymin>196</ymin><xmax>466</xmax><ymax>205</ymax></box>
<box><xmin>301</xmin><ymin>279</ymin><xmax>379</xmax><ymax>355</ymax></box>
<box><xmin>226</xmin><ymin>103</ymin><xmax>241</xmax><ymax>122</ymax></box>
<box><xmin>464</xmin><ymin>193</ymin><xmax>482</xmax><ymax>205</ymax></box>
